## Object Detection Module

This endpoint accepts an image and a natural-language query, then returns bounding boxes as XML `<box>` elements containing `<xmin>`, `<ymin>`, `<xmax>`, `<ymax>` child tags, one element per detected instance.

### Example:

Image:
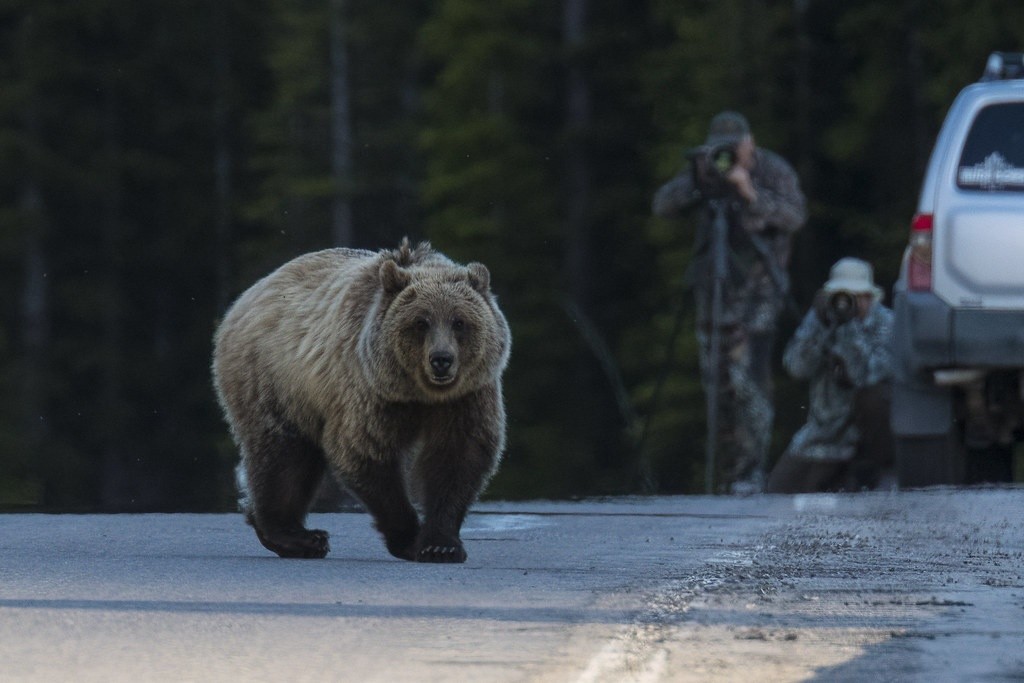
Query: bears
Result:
<box><xmin>211</xmin><ymin>236</ymin><xmax>511</xmax><ymax>561</ymax></box>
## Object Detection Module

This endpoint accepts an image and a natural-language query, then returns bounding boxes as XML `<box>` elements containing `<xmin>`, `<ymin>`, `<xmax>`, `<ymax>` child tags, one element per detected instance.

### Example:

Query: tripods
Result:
<box><xmin>634</xmin><ymin>173</ymin><xmax>798</xmax><ymax>498</ymax></box>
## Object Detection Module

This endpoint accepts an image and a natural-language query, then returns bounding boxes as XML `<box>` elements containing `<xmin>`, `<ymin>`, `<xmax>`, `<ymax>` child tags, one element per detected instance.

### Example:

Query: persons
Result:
<box><xmin>655</xmin><ymin>109</ymin><xmax>810</xmax><ymax>493</ymax></box>
<box><xmin>770</xmin><ymin>256</ymin><xmax>896</xmax><ymax>492</ymax></box>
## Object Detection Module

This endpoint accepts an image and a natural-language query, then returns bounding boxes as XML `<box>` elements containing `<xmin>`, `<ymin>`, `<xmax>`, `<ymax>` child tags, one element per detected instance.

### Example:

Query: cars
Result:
<box><xmin>889</xmin><ymin>48</ymin><xmax>1024</xmax><ymax>481</ymax></box>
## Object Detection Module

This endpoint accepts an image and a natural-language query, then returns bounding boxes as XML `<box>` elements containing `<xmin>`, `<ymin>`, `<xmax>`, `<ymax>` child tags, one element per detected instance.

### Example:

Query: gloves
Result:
<box><xmin>813</xmin><ymin>288</ymin><xmax>832</xmax><ymax>327</ymax></box>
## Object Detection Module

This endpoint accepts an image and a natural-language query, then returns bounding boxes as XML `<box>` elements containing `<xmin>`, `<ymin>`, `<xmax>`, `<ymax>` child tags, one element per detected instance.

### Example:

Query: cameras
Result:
<box><xmin>829</xmin><ymin>291</ymin><xmax>859</xmax><ymax>322</ymax></box>
<box><xmin>706</xmin><ymin>141</ymin><xmax>739</xmax><ymax>174</ymax></box>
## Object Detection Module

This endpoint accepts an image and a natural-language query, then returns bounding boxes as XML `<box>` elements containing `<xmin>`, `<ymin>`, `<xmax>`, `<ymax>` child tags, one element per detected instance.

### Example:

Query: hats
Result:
<box><xmin>705</xmin><ymin>110</ymin><xmax>749</xmax><ymax>147</ymax></box>
<box><xmin>823</xmin><ymin>257</ymin><xmax>881</xmax><ymax>303</ymax></box>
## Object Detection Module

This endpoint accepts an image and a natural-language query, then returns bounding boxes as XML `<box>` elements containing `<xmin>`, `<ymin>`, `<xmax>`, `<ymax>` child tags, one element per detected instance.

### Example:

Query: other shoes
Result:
<box><xmin>728</xmin><ymin>470</ymin><xmax>765</xmax><ymax>496</ymax></box>
<box><xmin>715</xmin><ymin>482</ymin><xmax>728</xmax><ymax>496</ymax></box>
